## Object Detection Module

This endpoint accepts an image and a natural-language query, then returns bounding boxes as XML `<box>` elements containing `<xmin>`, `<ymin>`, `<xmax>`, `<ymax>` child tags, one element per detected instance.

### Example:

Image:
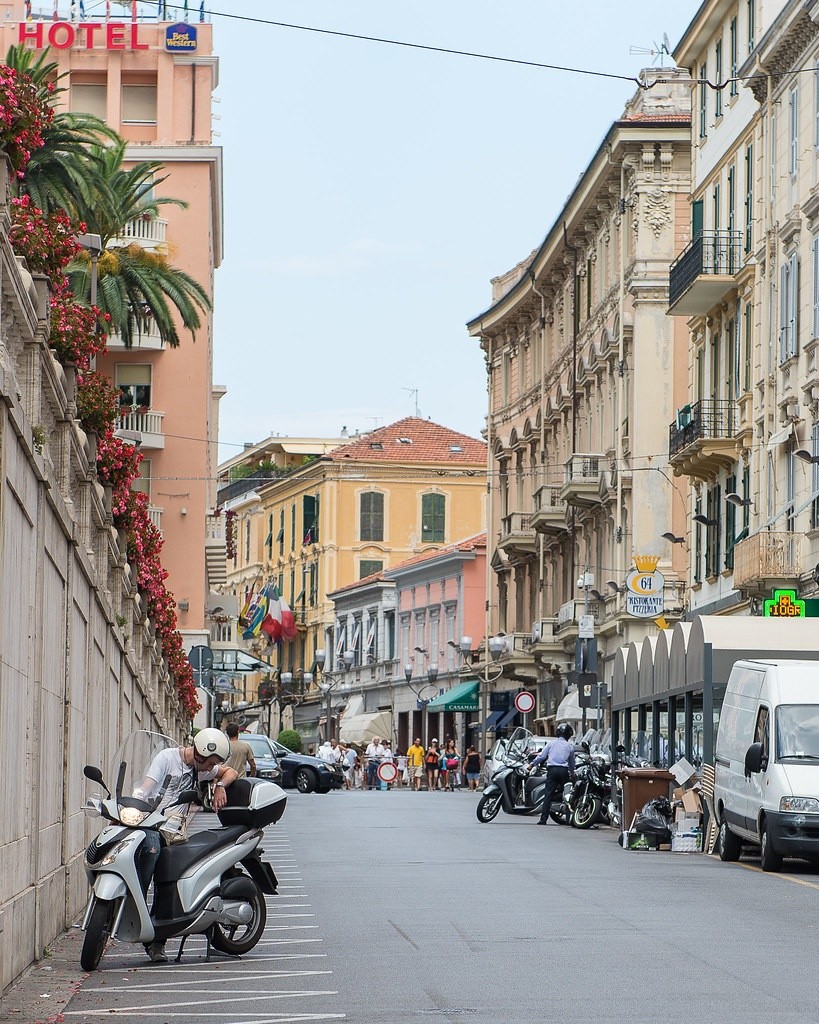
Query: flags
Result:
<box><xmin>239</xmin><ymin>584</ymin><xmax>298</xmax><ymax>641</ymax></box>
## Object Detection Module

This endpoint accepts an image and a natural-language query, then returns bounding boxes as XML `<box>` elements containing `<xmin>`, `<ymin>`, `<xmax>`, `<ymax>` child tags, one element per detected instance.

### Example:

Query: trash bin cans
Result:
<box><xmin>616</xmin><ymin>767</ymin><xmax>676</xmax><ymax>848</ymax></box>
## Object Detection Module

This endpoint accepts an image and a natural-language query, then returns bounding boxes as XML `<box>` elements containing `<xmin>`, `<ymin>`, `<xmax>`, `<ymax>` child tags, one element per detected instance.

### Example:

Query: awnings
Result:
<box><xmin>495</xmin><ymin>707</ymin><xmax>519</xmax><ymax>730</ymax></box>
<box><xmin>211</xmin><ymin>649</ymin><xmax>280</xmax><ymax>693</ymax></box>
<box><xmin>426</xmin><ymin>681</ymin><xmax>479</xmax><ymax>713</ymax></box>
<box><xmin>555</xmin><ymin>681</ymin><xmax>604</xmax><ymax>722</ymax></box>
<box><xmin>340</xmin><ymin>712</ymin><xmax>393</xmax><ymax>746</ymax></box>
<box><xmin>264</xmin><ymin>531</ymin><xmax>282</xmax><ymax>546</ymax></box>
<box><xmin>476</xmin><ymin>711</ymin><xmax>504</xmax><ymax>731</ymax></box>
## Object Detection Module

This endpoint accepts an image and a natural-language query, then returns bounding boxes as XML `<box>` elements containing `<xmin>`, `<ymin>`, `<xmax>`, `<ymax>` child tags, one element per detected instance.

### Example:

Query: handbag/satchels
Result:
<box><xmin>402</xmin><ymin>766</ymin><xmax>408</xmax><ymax>783</ymax></box>
<box><xmin>427</xmin><ymin>756</ymin><xmax>437</xmax><ymax>763</ymax></box>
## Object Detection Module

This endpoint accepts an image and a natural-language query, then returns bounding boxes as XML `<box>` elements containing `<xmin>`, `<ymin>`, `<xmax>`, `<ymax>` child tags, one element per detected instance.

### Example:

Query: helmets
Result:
<box><xmin>193</xmin><ymin>728</ymin><xmax>231</xmax><ymax>763</ymax></box>
<box><xmin>556</xmin><ymin>723</ymin><xmax>573</xmax><ymax>741</ymax></box>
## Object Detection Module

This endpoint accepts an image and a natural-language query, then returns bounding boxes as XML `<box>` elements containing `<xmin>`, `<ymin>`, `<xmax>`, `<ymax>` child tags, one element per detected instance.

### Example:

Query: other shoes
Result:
<box><xmin>148</xmin><ymin>943</ymin><xmax>168</xmax><ymax>962</ymax></box>
<box><xmin>537</xmin><ymin>822</ymin><xmax>546</xmax><ymax>825</ymax></box>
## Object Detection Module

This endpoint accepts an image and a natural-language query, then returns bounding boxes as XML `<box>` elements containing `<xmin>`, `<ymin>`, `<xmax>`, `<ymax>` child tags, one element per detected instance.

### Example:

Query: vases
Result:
<box><xmin>137</xmin><ymin>407</ymin><xmax>147</xmax><ymax>413</ymax></box>
<box><xmin>121</xmin><ymin>406</ymin><xmax>131</xmax><ymax>412</ymax></box>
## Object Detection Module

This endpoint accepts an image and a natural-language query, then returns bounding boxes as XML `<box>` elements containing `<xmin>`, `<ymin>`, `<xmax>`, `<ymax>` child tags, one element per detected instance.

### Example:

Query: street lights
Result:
<box><xmin>460</xmin><ymin>637</ymin><xmax>506</xmax><ymax>791</ymax></box>
<box><xmin>278</xmin><ymin>673</ymin><xmax>313</xmax><ymax>707</ymax></box>
<box><xmin>313</xmin><ymin>649</ymin><xmax>354</xmax><ymax>740</ymax></box>
<box><xmin>403</xmin><ymin>662</ymin><xmax>439</xmax><ymax>790</ymax></box>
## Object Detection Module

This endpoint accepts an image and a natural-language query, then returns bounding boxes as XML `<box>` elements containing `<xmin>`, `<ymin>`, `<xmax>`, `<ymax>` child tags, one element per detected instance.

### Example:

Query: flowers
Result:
<box><xmin>0</xmin><ymin>63</ymin><xmax>202</xmax><ymax>723</ymax></box>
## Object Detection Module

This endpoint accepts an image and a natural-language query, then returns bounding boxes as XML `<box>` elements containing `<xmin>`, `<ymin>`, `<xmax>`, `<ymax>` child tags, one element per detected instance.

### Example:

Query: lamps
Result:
<box><xmin>209</xmin><ymin>607</ymin><xmax>223</xmax><ymax>615</ymax></box>
<box><xmin>692</xmin><ymin>514</ymin><xmax>716</xmax><ymax>526</ymax></box>
<box><xmin>606</xmin><ymin>580</ymin><xmax>623</xmax><ymax>592</ymax></box>
<box><xmin>661</xmin><ymin>532</ymin><xmax>684</xmax><ymax>543</ymax></box>
<box><xmin>590</xmin><ymin>590</ymin><xmax>605</xmax><ymax>600</ymax></box>
<box><xmin>178</xmin><ymin>601</ymin><xmax>189</xmax><ymax>610</ymax></box>
<box><xmin>367</xmin><ymin>654</ymin><xmax>378</xmax><ymax>661</ymax></box>
<box><xmin>414</xmin><ymin>647</ymin><xmax>426</xmax><ymax>653</ymax></box>
<box><xmin>447</xmin><ymin>641</ymin><xmax>459</xmax><ymax>647</ymax></box>
<box><xmin>724</xmin><ymin>493</ymin><xmax>752</xmax><ymax>506</ymax></box>
<box><xmin>792</xmin><ymin>449</ymin><xmax>819</xmax><ymax>466</ymax></box>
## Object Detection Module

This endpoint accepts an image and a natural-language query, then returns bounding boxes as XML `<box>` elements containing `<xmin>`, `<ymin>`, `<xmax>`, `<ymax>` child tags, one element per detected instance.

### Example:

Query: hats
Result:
<box><xmin>381</xmin><ymin>740</ymin><xmax>387</xmax><ymax>744</ymax></box>
<box><xmin>432</xmin><ymin>739</ymin><xmax>437</xmax><ymax>742</ymax></box>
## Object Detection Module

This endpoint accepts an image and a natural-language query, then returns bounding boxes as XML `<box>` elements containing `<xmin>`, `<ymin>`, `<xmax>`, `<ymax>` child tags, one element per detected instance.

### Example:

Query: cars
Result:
<box><xmin>239</xmin><ymin>734</ymin><xmax>287</xmax><ymax>788</ymax></box>
<box><xmin>484</xmin><ymin>739</ymin><xmax>522</xmax><ymax>790</ymax></box>
<box><xmin>525</xmin><ymin>736</ymin><xmax>559</xmax><ymax>753</ymax></box>
<box><xmin>273</xmin><ymin>738</ymin><xmax>344</xmax><ymax>794</ymax></box>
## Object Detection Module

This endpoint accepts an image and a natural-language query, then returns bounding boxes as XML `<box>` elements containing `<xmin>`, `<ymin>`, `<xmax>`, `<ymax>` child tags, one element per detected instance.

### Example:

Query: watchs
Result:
<box><xmin>216</xmin><ymin>781</ymin><xmax>226</xmax><ymax>788</ymax></box>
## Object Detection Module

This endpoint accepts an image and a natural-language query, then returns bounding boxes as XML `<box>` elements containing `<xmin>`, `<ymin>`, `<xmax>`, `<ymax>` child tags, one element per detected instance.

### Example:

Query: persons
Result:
<box><xmin>529</xmin><ymin>723</ymin><xmax>576</xmax><ymax>825</ymax></box>
<box><xmin>462</xmin><ymin>745</ymin><xmax>483</xmax><ymax>792</ymax></box>
<box><xmin>137</xmin><ymin>729</ymin><xmax>239</xmax><ymax>962</ymax></box>
<box><xmin>308</xmin><ymin>735</ymin><xmax>393</xmax><ymax>790</ymax></box>
<box><xmin>406</xmin><ymin>737</ymin><xmax>426</xmax><ymax>791</ymax></box>
<box><xmin>212</xmin><ymin>723</ymin><xmax>256</xmax><ymax>794</ymax></box>
<box><xmin>393</xmin><ymin>748</ymin><xmax>406</xmax><ymax>787</ymax></box>
<box><xmin>425</xmin><ymin>737</ymin><xmax>461</xmax><ymax>791</ymax></box>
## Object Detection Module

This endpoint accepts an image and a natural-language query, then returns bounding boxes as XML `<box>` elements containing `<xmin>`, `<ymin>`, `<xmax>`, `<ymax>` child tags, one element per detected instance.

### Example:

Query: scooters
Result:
<box><xmin>78</xmin><ymin>729</ymin><xmax>288</xmax><ymax>971</ymax></box>
<box><xmin>477</xmin><ymin>727</ymin><xmax>657</xmax><ymax>829</ymax></box>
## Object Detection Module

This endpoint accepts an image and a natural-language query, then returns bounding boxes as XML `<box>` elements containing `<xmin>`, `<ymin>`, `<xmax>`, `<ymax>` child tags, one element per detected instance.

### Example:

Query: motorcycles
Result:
<box><xmin>200</xmin><ymin>778</ymin><xmax>218</xmax><ymax>811</ymax></box>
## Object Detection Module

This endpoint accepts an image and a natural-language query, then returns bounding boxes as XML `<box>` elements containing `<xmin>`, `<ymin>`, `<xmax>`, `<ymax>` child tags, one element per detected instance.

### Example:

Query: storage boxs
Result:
<box><xmin>628</xmin><ymin>833</ymin><xmax>657</xmax><ymax>848</ymax></box>
<box><xmin>659</xmin><ymin>757</ymin><xmax>704</xmax><ymax>850</ymax></box>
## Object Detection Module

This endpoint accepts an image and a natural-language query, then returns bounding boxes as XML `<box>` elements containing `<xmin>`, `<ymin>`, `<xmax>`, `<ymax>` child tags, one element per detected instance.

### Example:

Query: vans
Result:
<box><xmin>713</xmin><ymin>658</ymin><xmax>819</xmax><ymax>872</ymax></box>
